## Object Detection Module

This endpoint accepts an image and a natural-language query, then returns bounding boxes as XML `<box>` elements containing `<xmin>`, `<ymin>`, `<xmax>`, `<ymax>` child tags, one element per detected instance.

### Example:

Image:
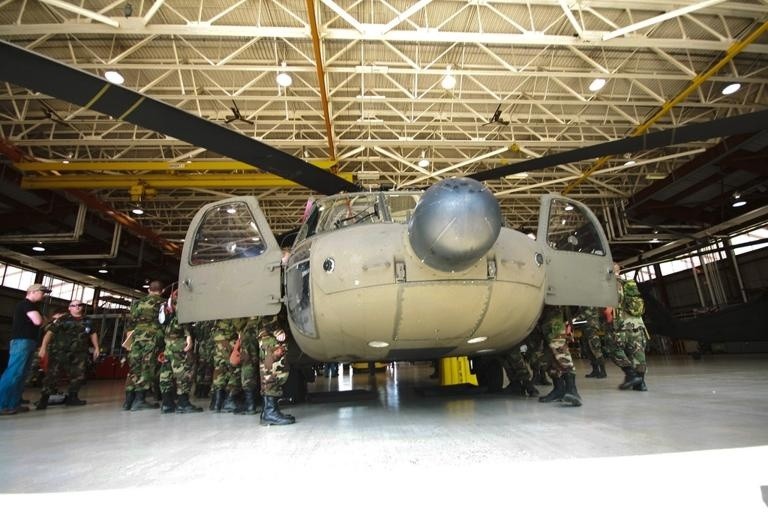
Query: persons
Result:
<box><xmin>121</xmin><ymin>280</ymin><xmax>339</xmax><ymax>425</ymax></box>
<box><xmin>35</xmin><ymin>300</ymin><xmax>100</xmax><ymax>409</ymax></box>
<box><xmin>0</xmin><ymin>283</ymin><xmax>52</xmax><ymax>415</ymax></box>
<box><xmin>429</xmin><ymin>262</ymin><xmax>650</xmax><ymax>406</ymax></box>
<box><xmin>27</xmin><ymin>309</ymin><xmax>64</xmax><ymax>396</ymax></box>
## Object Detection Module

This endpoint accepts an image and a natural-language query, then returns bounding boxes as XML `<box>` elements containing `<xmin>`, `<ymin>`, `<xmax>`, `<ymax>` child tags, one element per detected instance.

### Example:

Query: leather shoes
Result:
<box><xmin>0</xmin><ymin>398</ymin><xmax>30</xmax><ymax>415</ymax></box>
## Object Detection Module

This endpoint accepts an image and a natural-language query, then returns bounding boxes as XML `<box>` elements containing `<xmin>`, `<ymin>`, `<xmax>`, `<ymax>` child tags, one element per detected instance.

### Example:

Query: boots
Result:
<box><xmin>584</xmin><ymin>362</ymin><xmax>607</xmax><ymax>379</ymax></box>
<box><xmin>119</xmin><ymin>389</ymin><xmax>258</xmax><ymax>416</ymax></box>
<box><xmin>561</xmin><ymin>372</ymin><xmax>584</xmax><ymax>407</ymax></box>
<box><xmin>259</xmin><ymin>394</ymin><xmax>296</xmax><ymax>426</ymax></box>
<box><xmin>64</xmin><ymin>391</ymin><xmax>88</xmax><ymax>407</ymax></box>
<box><xmin>539</xmin><ymin>376</ymin><xmax>566</xmax><ymax>404</ymax></box>
<box><xmin>497</xmin><ymin>369</ymin><xmax>553</xmax><ymax>398</ymax></box>
<box><xmin>618</xmin><ymin>365</ymin><xmax>648</xmax><ymax>392</ymax></box>
<box><xmin>34</xmin><ymin>395</ymin><xmax>50</xmax><ymax>411</ymax></box>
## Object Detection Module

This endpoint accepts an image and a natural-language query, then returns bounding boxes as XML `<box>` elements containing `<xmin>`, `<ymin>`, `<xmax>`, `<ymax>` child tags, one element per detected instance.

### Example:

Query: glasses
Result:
<box><xmin>69</xmin><ymin>303</ymin><xmax>85</xmax><ymax>308</ymax></box>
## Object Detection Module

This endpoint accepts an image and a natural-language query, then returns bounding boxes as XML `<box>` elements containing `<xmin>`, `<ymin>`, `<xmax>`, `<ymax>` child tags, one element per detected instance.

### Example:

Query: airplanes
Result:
<box><xmin>638</xmin><ymin>287</ymin><xmax>768</xmax><ymax>342</ymax></box>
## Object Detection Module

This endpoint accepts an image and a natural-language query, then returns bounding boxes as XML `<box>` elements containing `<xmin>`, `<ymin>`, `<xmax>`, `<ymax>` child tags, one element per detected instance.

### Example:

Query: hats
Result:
<box><xmin>26</xmin><ymin>283</ymin><xmax>53</xmax><ymax>294</ymax></box>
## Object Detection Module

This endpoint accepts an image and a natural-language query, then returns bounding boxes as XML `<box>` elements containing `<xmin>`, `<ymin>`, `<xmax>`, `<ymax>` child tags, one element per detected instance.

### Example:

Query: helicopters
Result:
<box><xmin>0</xmin><ymin>42</ymin><xmax>768</xmax><ymax>408</ymax></box>
